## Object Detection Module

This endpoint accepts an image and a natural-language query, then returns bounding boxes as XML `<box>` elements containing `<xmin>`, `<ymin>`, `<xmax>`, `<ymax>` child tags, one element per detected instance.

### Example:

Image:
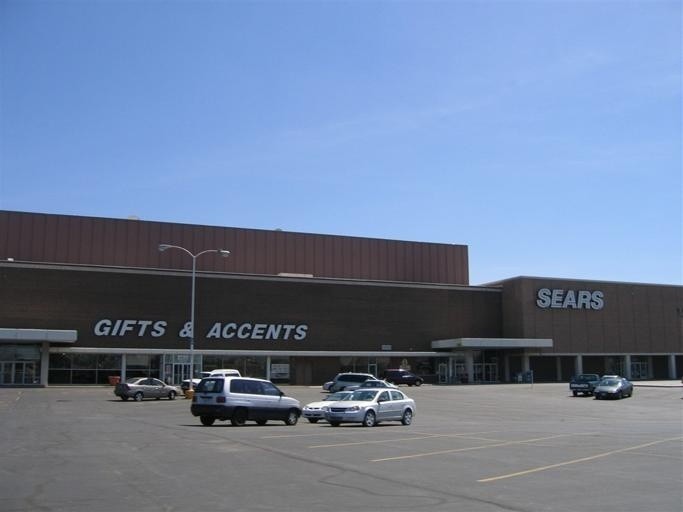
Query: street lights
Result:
<box><xmin>158</xmin><ymin>243</ymin><xmax>230</xmax><ymax>399</ymax></box>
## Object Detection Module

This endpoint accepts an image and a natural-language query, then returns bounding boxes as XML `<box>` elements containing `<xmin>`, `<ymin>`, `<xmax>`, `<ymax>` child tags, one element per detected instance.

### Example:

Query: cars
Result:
<box><xmin>113</xmin><ymin>376</ymin><xmax>180</xmax><ymax>401</ymax></box>
<box><xmin>180</xmin><ymin>368</ymin><xmax>423</xmax><ymax>426</ymax></box>
<box><xmin>570</xmin><ymin>374</ymin><xmax>633</xmax><ymax>398</ymax></box>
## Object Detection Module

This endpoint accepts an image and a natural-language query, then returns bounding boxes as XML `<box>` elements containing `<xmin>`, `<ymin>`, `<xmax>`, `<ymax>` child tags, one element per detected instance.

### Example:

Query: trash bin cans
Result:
<box><xmin>461</xmin><ymin>373</ymin><xmax>469</xmax><ymax>384</ymax></box>
<box><xmin>109</xmin><ymin>376</ymin><xmax>121</xmax><ymax>385</ymax></box>
